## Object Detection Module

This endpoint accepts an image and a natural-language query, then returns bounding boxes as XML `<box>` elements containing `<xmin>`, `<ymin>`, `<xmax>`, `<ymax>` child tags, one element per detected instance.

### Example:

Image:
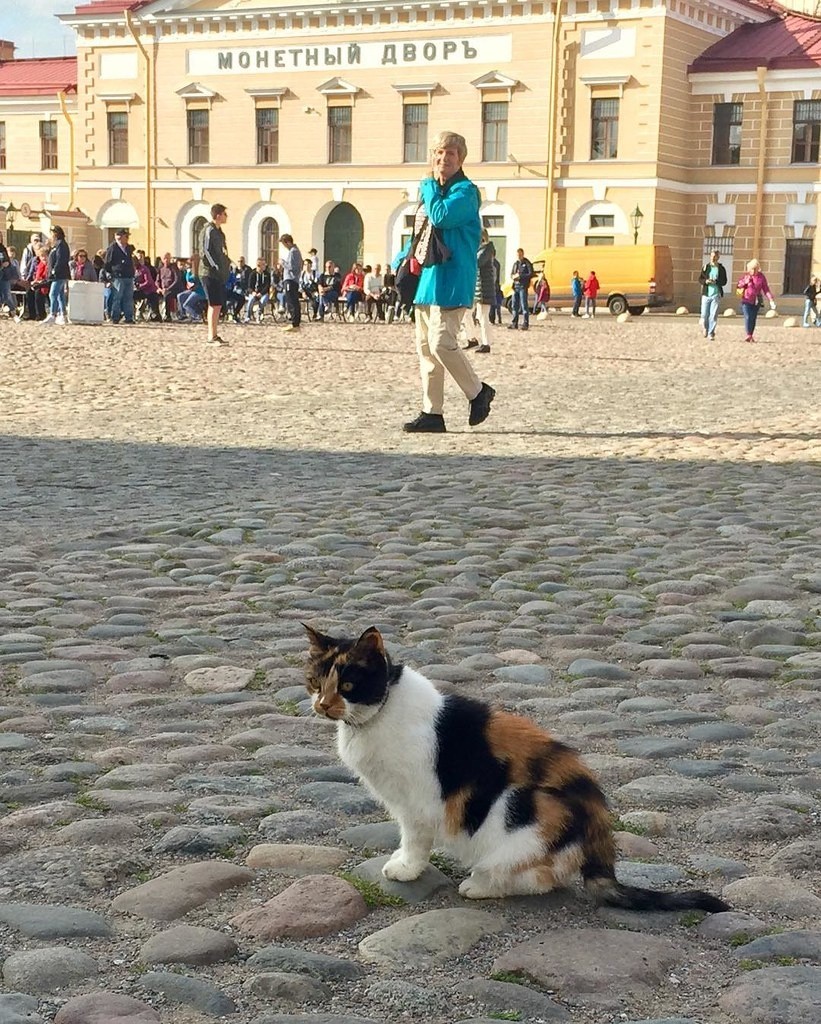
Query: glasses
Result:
<box><xmin>78</xmin><ymin>255</ymin><xmax>86</xmax><ymax>258</ymax></box>
<box><xmin>121</xmin><ymin>236</ymin><xmax>130</xmax><ymax>238</ymax></box>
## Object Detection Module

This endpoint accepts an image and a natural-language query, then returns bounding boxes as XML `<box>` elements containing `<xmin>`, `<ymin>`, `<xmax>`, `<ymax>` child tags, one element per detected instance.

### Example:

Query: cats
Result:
<box><xmin>301</xmin><ymin>621</ymin><xmax>733</xmax><ymax>914</ymax></box>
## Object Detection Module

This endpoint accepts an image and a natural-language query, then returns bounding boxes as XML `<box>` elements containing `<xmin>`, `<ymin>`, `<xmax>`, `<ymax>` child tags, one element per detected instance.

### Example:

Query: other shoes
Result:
<box><xmin>498</xmin><ymin>322</ymin><xmax>502</xmax><ymax>325</ymax></box>
<box><xmin>123</xmin><ymin>319</ymin><xmax>136</xmax><ymax>324</ymax></box>
<box><xmin>746</xmin><ymin>335</ymin><xmax>755</xmax><ymax>343</ymax></box>
<box><xmin>207</xmin><ymin>337</ymin><xmax>229</xmax><ymax>346</ymax></box>
<box><xmin>468</xmin><ymin>384</ymin><xmax>496</xmax><ymax>426</ymax></box>
<box><xmin>475</xmin><ymin>345</ymin><xmax>492</xmax><ymax>353</ymax></box>
<box><xmin>508</xmin><ymin>325</ymin><xmax>519</xmax><ymax>329</ymax></box>
<box><xmin>403</xmin><ymin>413</ymin><xmax>448</xmax><ymax>432</ymax></box>
<box><xmin>803</xmin><ymin>322</ymin><xmax>810</xmax><ymax>327</ymax></box>
<box><xmin>573</xmin><ymin>312</ymin><xmax>582</xmax><ymax>317</ymax></box>
<box><xmin>112</xmin><ymin>320</ymin><xmax>119</xmax><ymax>324</ymax></box>
<box><xmin>582</xmin><ymin>314</ymin><xmax>590</xmax><ymax>318</ymax></box>
<box><xmin>709</xmin><ymin>335</ymin><xmax>715</xmax><ymax>341</ymax></box>
<box><xmin>703</xmin><ymin>329</ymin><xmax>708</xmax><ymax>337</ymax></box>
<box><xmin>489</xmin><ymin>321</ymin><xmax>494</xmax><ymax>326</ymax></box>
<box><xmin>591</xmin><ymin>315</ymin><xmax>596</xmax><ymax>319</ymax></box>
<box><xmin>282</xmin><ymin>324</ymin><xmax>300</xmax><ymax>333</ymax></box>
<box><xmin>522</xmin><ymin>326</ymin><xmax>529</xmax><ymax>330</ymax></box>
<box><xmin>13</xmin><ymin>315</ymin><xmax>22</xmax><ymax>323</ymax></box>
<box><xmin>463</xmin><ymin>340</ymin><xmax>480</xmax><ymax>351</ymax></box>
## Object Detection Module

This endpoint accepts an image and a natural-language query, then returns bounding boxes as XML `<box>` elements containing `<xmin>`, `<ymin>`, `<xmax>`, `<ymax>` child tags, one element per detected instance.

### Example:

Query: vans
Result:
<box><xmin>500</xmin><ymin>245</ymin><xmax>675</xmax><ymax>316</ymax></box>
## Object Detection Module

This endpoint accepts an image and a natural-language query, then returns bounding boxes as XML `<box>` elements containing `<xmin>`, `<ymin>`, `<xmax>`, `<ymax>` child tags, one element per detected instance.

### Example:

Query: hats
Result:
<box><xmin>31</xmin><ymin>234</ymin><xmax>40</xmax><ymax>241</ymax></box>
<box><xmin>50</xmin><ymin>226</ymin><xmax>63</xmax><ymax>233</ymax></box>
<box><xmin>117</xmin><ymin>230</ymin><xmax>132</xmax><ymax>235</ymax></box>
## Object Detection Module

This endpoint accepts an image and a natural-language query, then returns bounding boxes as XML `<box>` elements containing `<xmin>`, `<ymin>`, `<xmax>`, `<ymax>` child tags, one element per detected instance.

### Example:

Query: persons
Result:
<box><xmin>801</xmin><ymin>277</ymin><xmax>821</xmax><ymax>328</ymax></box>
<box><xmin>0</xmin><ymin>131</ymin><xmax>599</xmax><ymax>433</ymax></box>
<box><xmin>699</xmin><ymin>249</ymin><xmax>727</xmax><ymax>340</ymax></box>
<box><xmin>736</xmin><ymin>257</ymin><xmax>777</xmax><ymax>343</ymax></box>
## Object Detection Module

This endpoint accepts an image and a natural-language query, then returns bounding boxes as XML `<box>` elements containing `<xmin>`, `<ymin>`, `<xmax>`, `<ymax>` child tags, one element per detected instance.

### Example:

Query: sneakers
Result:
<box><xmin>56</xmin><ymin>316</ymin><xmax>68</xmax><ymax>326</ymax></box>
<box><xmin>40</xmin><ymin>316</ymin><xmax>56</xmax><ymax>325</ymax></box>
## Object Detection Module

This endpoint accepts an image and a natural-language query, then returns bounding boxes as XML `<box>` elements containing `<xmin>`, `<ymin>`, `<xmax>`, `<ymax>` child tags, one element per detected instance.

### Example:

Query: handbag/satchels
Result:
<box><xmin>753</xmin><ymin>295</ymin><xmax>765</xmax><ymax>308</ymax></box>
<box><xmin>413</xmin><ymin>173</ymin><xmax>461</xmax><ymax>267</ymax></box>
<box><xmin>396</xmin><ymin>246</ymin><xmax>418</xmax><ymax>322</ymax></box>
<box><xmin>736</xmin><ymin>288</ymin><xmax>745</xmax><ymax>298</ymax></box>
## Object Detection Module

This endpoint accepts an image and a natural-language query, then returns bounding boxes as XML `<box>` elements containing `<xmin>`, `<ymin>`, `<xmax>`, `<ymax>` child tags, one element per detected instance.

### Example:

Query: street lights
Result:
<box><xmin>630</xmin><ymin>204</ymin><xmax>645</xmax><ymax>244</ymax></box>
